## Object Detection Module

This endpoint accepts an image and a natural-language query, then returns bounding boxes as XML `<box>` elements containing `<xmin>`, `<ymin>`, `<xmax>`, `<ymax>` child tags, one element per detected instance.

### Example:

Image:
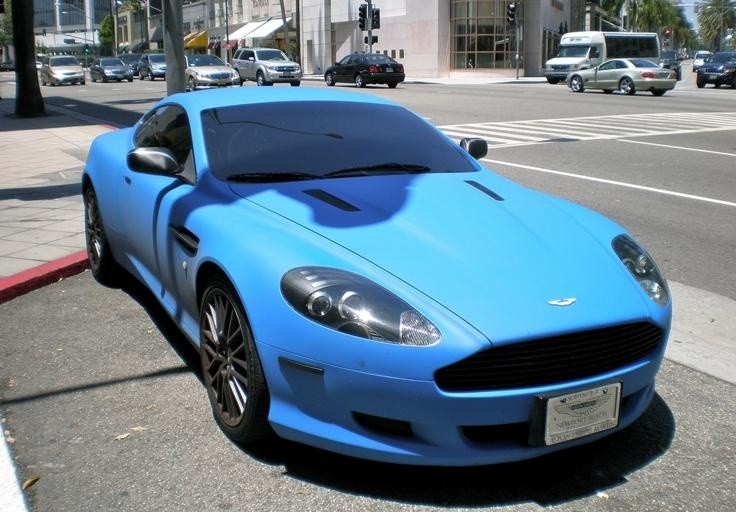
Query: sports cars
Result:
<box><xmin>81</xmin><ymin>86</ymin><xmax>673</xmax><ymax>468</ymax></box>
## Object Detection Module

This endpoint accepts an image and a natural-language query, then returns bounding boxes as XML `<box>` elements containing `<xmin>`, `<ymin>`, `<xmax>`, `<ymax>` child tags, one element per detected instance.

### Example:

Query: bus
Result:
<box><xmin>543</xmin><ymin>31</ymin><xmax>661</xmax><ymax>85</ymax></box>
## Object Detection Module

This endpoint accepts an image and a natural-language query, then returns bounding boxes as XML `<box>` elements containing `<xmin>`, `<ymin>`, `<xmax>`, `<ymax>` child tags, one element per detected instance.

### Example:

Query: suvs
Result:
<box><xmin>697</xmin><ymin>52</ymin><xmax>736</xmax><ymax>89</ymax></box>
<box><xmin>231</xmin><ymin>47</ymin><xmax>303</xmax><ymax>87</ymax></box>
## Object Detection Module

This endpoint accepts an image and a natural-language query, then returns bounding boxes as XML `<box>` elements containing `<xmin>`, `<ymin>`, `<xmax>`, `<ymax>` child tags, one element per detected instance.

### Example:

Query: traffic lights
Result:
<box><xmin>42</xmin><ymin>29</ymin><xmax>46</xmax><ymax>38</ymax></box>
<box><xmin>372</xmin><ymin>8</ymin><xmax>380</xmax><ymax>29</ymax></box>
<box><xmin>666</xmin><ymin>30</ymin><xmax>670</xmax><ymax>39</ymax></box>
<box><xmin>694</xmin><ymin>2</ymin><xmax>699</xmax><ymax>14</ymax></box>
<box><xmin>507</xmin><ymin>4</ymin><xmax>515</xmax><ymax>27</ymax></box>
<box><xmin>371</xmin><ymin>36</ymin><xmax>378</xmax><ymax>44</ymax></box>
<box><xmin>84</xmin><ymin>44</ymin><xmax>88</xmax><ymax>53</ymax></box>
<box><xmin>364</xmin><ymin>37</ymin><xmax>368</xmax><ymax>45</ymax></box>
<box><xmin>666</xmin><ymin>42</ymin><xmax>670</xmax><ymax>47</ymax></box>
<box><xmin>358</xmin><ymin>4</ymin><xmax>367</xmax><ymax>32</ymax></box>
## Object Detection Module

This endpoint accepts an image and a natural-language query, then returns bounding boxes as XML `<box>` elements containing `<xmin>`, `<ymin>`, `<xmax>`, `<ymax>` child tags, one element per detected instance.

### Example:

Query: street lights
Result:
<box><xmin>706</xmin><ymin>6</ymin><xmax>736</xmax><ymax>52</ymax></box>
<box><xmin>116</xmin><ymin>1</ymin><xmax>144</xmax><ymax>54</ymax></box>
<box><xmin>53</xmin><ymin>3</ymin><xmax>95</xmax><ymax>63</ymax></box>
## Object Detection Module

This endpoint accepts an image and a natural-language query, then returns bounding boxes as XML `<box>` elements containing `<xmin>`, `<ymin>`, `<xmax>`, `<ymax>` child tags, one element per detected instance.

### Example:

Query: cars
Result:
<box><xmin>659</xmin><ymin>48</ymin><xmax>714</xmax><ymax>82</ymax></box>
<box><xmin>35</xmin><ymin>53</ymin><xmax>166</xmax><ymax>87</ymax></box>
<box><xmin>324</xmin><ymin>53</ymin><xmax>405</xmax><ymax>89</ymax></box>
<box><xmin>183</xmin><ymin>54</ymin><xmax>241</xmax><ymax>92</ymax></box>
<box><xmin>457</xmin><ymin>50</ymin><xmax>522</xmax><ymax>69</ymax></box>
<box><xmin>566</xmin><ymin>57</ymin><xmax>677</xmax><ymax>97</ymax></box>
